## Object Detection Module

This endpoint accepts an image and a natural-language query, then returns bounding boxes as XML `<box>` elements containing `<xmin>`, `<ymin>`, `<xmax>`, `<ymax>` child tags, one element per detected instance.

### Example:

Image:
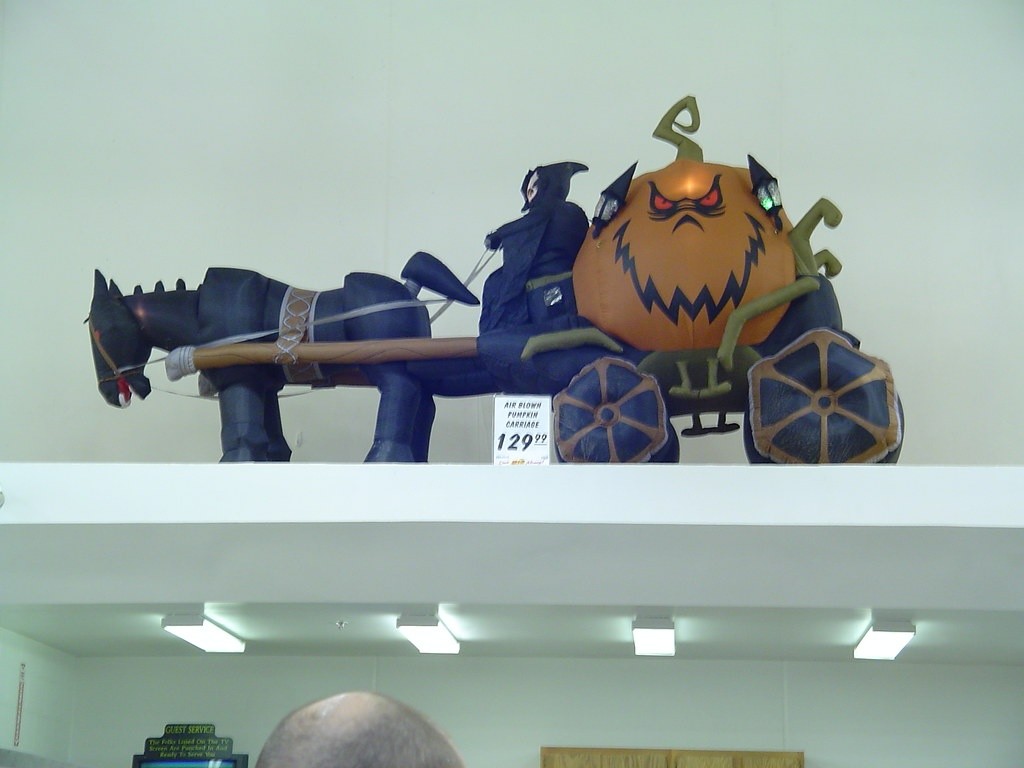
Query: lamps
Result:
<box><xmin>853</xmin><ymin>621</ymin><xmax>916</xmax><ymax>659</ymax></box>
<box><xmin>396</xmin><ymin>615</ymin><xmax>460</xmax><ymax>656</ymax></box>
<box><xmin>632</xmin><ymin>618</ymin><xmax>676</xmax><ymax>657</ymax></box>
<box><xmin>161</xmin><ymin>616</ymin><xmax>247</xmax><ymax>653</ymax></box>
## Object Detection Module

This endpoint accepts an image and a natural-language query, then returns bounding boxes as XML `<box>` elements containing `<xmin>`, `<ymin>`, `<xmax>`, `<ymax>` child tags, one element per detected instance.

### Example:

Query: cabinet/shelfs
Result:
<box><xmin>540</xmin><ymin>746</ymin><xmax>804</xmax><ymax>768</ymax></box>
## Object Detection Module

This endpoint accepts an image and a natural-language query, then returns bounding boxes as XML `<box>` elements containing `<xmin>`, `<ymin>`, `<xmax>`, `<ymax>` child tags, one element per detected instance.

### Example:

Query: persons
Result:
<box><xmin>254</xmin><ymin>690</ymin><xmax>464</xmax><ymax>768</ymax></box>
